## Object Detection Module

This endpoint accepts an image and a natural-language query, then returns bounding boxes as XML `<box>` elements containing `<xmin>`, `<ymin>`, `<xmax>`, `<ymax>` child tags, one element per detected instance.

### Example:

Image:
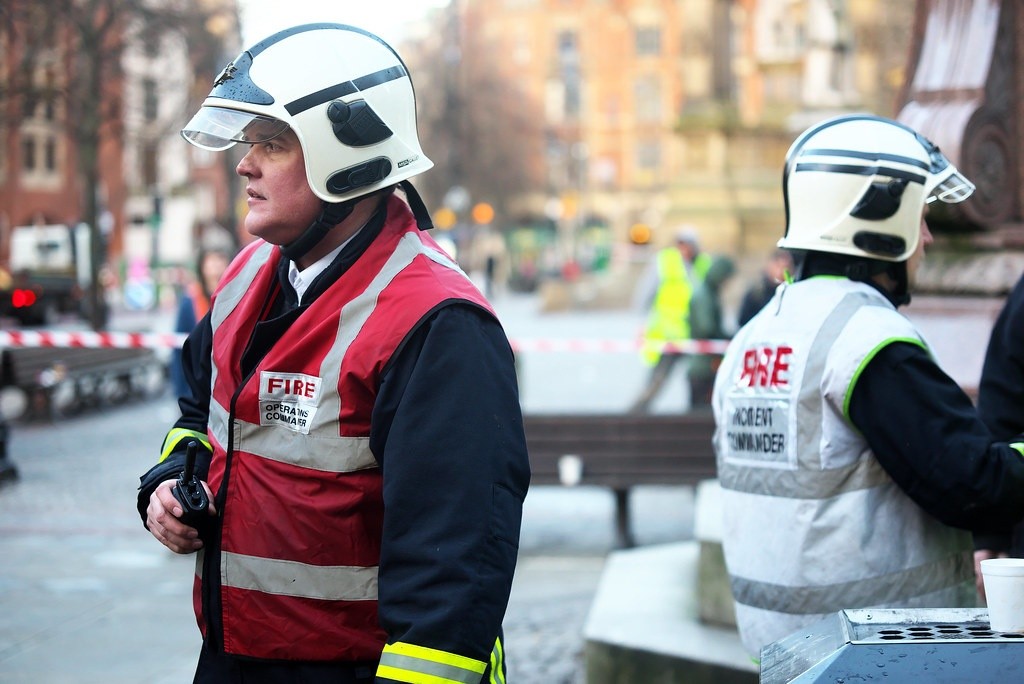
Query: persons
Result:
<box><xmin>169</xmin><ymin>245</ymin><xmax>231</xmax><ymax>402</ymax></box>
<box><xmin>711</xmin><ymin>115</ymin><xmax>1023</xmax><ymax>684</ymax></box>
<box><xmin>680</xmin><ymin>256</ymin><xmax>736</xmax><ymax>418</ymax></box>
<box><xmin>632</xmin><ymin>248</ymin><xmax>691</xmax><ymax>415</ymax></box>
<box><xmin>740</xmin><ymin>246</ymin><xmax>796</xmax><ymax>326</ymax></box>
<box><xmin>135</xmin><ymin>23</ymin><xmax>533</xmax><ymax>684</ymax></box>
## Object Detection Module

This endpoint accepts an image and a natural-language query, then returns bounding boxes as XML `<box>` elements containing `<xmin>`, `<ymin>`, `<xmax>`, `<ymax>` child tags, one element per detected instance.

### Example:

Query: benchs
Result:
<box><xmin>516</xmin><ymin>410</ymin><xmax>720</xmax><ymax>547</ymax></box>
<box><xmin>0</xmin><ymin>343</ymin><xmax>154</xmax><ymax>422</ymax></box>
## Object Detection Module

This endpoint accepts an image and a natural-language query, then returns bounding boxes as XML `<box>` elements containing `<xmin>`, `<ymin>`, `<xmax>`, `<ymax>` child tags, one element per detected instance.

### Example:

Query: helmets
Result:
<box><xmin>777</xmin><ymin>115</ymin><xmax>976</xmax><ymax>262</ymax></box>
<box><xmin>181</xmin><ymin>23</ymin><xmax>437</xmax><ymax>203</ymax></box>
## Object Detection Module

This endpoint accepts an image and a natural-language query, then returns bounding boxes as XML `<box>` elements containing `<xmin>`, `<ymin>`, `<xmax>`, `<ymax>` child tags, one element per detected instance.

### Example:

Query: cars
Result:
<box><xmin>5</xmin><ymin>266</ymin><xmax>111</xmax><ymax>330</ymax></box>
